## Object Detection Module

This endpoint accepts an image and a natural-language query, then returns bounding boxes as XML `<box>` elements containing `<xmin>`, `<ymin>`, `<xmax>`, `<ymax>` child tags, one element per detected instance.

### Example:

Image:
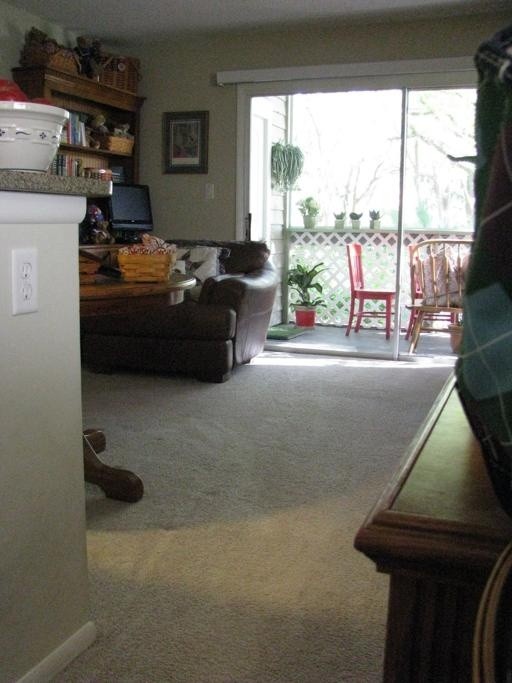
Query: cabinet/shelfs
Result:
<box><xmin>10</xmin><ymin>66</ymin><xmax>146</xmax><ymax>183</ymax></box>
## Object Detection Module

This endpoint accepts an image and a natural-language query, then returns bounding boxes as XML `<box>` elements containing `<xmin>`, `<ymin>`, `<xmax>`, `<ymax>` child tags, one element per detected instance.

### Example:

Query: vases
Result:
<box><xmin>0</xmin><ymin>100</ymin><xmax>70</xmax><ymax>173</ymax></box>
<box><xmin>303</xmin><ymin>217</ymin><xmax>316</xmax><ymax>229</ymax></box>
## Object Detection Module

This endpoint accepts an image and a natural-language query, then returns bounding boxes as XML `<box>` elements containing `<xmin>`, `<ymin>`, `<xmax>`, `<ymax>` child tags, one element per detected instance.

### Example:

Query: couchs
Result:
<box><xmin>81</xmin><ymin>256</ymin><xmax>280</xmax><ymax>383</ymax></box>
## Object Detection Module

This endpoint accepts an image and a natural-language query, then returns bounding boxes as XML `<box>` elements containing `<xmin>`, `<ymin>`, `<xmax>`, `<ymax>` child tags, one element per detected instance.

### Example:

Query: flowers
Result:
<box><xmin>296</xmin><ymin>197</ymin><xmax>321</xmax><ymax>216</ymax></box>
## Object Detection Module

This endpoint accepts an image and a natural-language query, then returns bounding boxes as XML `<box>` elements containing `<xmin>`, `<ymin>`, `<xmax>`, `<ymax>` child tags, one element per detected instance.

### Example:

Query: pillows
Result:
<box><xmin>164</xmin><ymin>239</ymin><xmax>271</xmax><ymax>307</ymax></box>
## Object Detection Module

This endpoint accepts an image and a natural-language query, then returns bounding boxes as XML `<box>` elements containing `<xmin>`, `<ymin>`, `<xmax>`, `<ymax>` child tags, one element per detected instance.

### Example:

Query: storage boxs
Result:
<box><xmin>118</xmin><ymin>255</ymin><xmax>173</xmax><ymax>284</ymax></box>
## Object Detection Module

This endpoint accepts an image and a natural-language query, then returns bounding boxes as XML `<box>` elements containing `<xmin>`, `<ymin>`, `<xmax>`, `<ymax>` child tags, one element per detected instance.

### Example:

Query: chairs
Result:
<box><xmin>404</xmin><ymin>239</ymin><xmax>474</xmax><ymax>354</ymax></box>
<box><xmin>346</xmin><ymin>242</ymin><xmax>397</xmax><ymax>340</ymax></box>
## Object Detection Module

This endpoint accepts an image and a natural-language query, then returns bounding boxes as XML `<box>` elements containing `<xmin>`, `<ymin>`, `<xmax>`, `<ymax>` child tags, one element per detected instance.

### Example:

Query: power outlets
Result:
<box><xmin>11</xmin><ymin>246</ymin><xmax>40</xmax><ymax>314</ymax></box>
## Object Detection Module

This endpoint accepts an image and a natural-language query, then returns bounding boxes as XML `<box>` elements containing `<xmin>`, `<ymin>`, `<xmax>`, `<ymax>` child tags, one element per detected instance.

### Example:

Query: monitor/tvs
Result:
<box><xmin>108</xmin><ymin>183</ymin><xmax>153</xmax><ymax>244</ymax></box>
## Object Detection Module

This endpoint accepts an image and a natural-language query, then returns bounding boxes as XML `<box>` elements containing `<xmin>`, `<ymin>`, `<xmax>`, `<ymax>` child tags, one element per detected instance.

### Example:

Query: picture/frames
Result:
<box><xmin>161</xmin><ymin>111</ymin><xmax>209</xmax><ymax>175</ymax></box>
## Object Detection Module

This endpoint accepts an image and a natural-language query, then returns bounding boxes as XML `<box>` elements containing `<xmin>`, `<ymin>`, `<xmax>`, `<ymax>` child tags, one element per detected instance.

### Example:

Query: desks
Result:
<box><xmin>80</xmin><ymin>276</ymin><xmax>197</xmax><ymax>317</ymax></box>
<box><xmin>354</xmin><ymin>367</ymin><xmax>510</xmax><ymax>683</ymax></box>
<box><xmin>1</xmin><ymin>171</ymin><xmax>116</xmax><ymax>683</ymax></box>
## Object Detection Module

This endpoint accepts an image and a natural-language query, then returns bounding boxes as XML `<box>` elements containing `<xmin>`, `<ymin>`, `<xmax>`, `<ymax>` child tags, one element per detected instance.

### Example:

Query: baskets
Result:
<box><xmin>25</xmin><ymin>46</ymin><xmax>79</xmax><ymax>74</ymax></box>
<box><xmin>100</xmin><ymin>56</ymin><xmax>139</xmax><ymax>93</ymax></box>
<box><xmin>91</xmin><ymin>134</ymin><xmax>134</xmax><ymax>154</ymax></box>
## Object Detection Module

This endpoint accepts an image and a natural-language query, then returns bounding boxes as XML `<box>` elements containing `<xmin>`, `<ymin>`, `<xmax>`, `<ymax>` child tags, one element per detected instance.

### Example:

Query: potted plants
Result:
<box><xmin>369</xmin><ymin>210</ymin><xmax>384</xmax><ymax>229</ymax></box>
<box><xmin>349</xmin><ymin>212</ymin><xmax>364</xmax><ymax>230</ymax></box>
<box><xmin>333</xmin><ymin>210</ymin><xmax>346</xmax><ymax>229</ymax></box>
<box><xmin>287</xmin><ymin>262</ymin><xmax>334</xmax><ymax>330</ymax></box>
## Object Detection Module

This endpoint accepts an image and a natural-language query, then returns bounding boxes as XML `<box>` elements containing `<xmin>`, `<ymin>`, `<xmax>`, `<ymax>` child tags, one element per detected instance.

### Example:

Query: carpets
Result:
<box><xmin>267</xmin><ymin>326</ymin><xmax>304</xmax><ymax>340</ymax></box>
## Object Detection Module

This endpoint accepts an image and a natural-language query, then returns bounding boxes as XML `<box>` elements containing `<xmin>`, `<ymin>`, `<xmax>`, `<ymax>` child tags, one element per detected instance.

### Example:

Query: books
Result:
<box><xmin>50</xmin><ymin>111</ymin><xmax>87</xmax><ymax>177</ymax></box>
